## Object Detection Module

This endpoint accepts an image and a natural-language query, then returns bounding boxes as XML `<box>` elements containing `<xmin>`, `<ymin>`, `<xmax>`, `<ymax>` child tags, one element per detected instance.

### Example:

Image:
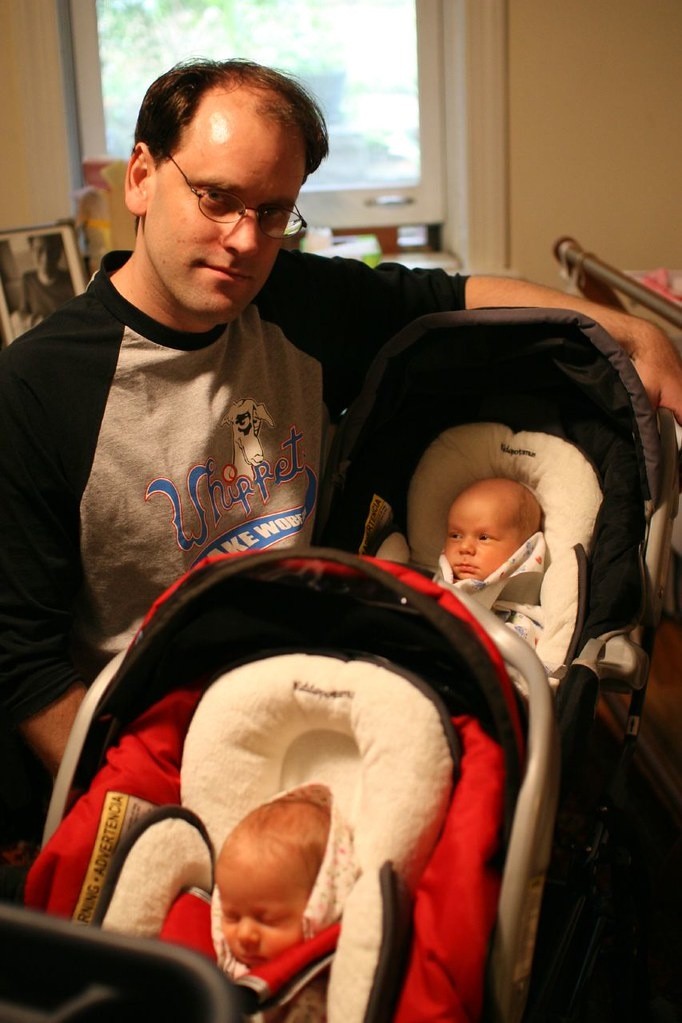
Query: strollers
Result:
<box><xmin>1</xmin><ymin>548</ymin><xmax>562</xmax><ymax>1023</ymax></box>
<box><xmin>308</xmin><ymin>302</ymin><xmax>679</xmax><ymax>1023</ymax></box>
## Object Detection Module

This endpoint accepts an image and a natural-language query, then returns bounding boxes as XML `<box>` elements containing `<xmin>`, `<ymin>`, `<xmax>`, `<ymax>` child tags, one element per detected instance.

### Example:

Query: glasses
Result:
<box><xmin>165</xmin><ymin>152</ymin><xmax>308</xmax><ymax>240</ymax></box>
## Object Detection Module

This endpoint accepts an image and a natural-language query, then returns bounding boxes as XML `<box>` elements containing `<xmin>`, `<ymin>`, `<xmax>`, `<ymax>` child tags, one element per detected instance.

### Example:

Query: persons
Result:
<box><xmin>429</xmin><ymin>476</ymin><xmax>546</xmax><ymax>651</ymax></box>
<box><xmin>208</xmin><ymin>782</ymin><xmax>362</xmax><ymax>982</ymax></box>
<box><xmin>0</xmin><ymin>59</ymin><xmax>682</xmax><ymax>786</ymax></box>
<box><xmin>18</xmin><ymin>234</ymin><xmax>75</xmax><ymax>329</ymax></box>
